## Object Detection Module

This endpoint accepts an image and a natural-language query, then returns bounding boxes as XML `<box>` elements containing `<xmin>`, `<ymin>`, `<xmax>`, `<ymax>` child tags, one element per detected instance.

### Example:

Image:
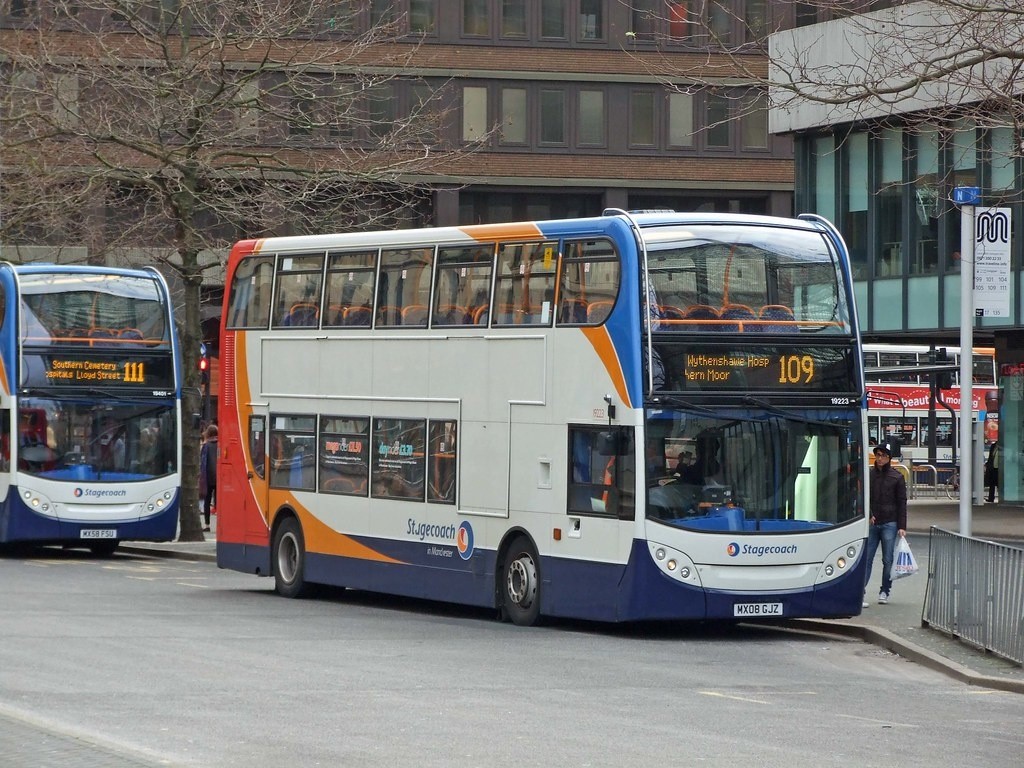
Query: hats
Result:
<box><xmin>873</xmin><ymin>443</ymin><xmax>891</xmax><ymax>455</ymax></box>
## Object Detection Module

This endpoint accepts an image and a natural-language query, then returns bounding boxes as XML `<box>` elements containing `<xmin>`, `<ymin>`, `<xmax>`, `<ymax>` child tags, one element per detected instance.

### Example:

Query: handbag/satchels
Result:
<box><xmin>889</xmin><ymin>536</ymin><xmax>919</xmax><ymax>581</ymax></box>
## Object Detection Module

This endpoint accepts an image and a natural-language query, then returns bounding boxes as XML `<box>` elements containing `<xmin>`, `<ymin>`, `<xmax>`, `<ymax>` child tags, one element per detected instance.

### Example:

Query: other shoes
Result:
<box><xmin>986</xmin><ymin>499</ymin><xmax>994</xmax><ymax>502</ymax></box>
<box><xmin>202</xmin><ymin>527</ymin><xmax>210</xmax><ymax>532</ymax></box>
<box><xmin>211</xmin><ymin>508</ymin><xmax>217</xmax><ymax>515</ymax></box>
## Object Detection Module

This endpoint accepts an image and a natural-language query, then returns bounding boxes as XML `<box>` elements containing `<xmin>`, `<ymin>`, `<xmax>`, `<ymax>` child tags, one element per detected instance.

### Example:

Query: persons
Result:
<box><xmin>863</xmin><ymin>444</ymin><xmax>906</xmax><ymax>605</ymax></box>
<box><xmin>21</xmin><ymin>420</ymin><xmax>45</xmax><ymax>449</ymax></box>
<box><xmin>985</xmin><ymin>443</ymin><xmax>998</xmax><ymax>503</ymax></box>
<box><xmin>198</xmin><ymin>424</ymin><xmax>219</xmax><ymax>531</ymax></box>
<box><xmin>601</xmin><ymin>453</ymin><xmax>667</xmax><ymax>516</ymax></box>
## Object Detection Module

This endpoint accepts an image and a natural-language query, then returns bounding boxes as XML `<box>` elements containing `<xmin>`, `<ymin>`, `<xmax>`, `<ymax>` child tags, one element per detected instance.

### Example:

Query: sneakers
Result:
<box><xmin>862</xmin><ymin>602</ymin><xmax>869</xmax><ymax>608</ymax></box>
<box><xmin>878</xmin><ymin>592</ymin><xmax>888</xmax><ymax>603</ymax></box>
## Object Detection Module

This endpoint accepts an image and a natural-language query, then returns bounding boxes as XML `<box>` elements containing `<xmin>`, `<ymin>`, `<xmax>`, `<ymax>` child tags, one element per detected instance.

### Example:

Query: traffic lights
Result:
<box><xmin>937</xmin><ymin>347</ymin><xmax>955</xmax><ymax>390</ymax></box>
<box><xmin>199</xmin><ymin>343</ymin><xmax>210</xmax><ymax>385</ymax></box>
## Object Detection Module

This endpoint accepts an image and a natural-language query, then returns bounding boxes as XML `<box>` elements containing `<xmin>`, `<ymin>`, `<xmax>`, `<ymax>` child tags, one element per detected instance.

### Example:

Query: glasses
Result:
<box><xmin>875</xmin><ymin>453</ymin><xmax>890</xmax><ymax>458</ymax></box>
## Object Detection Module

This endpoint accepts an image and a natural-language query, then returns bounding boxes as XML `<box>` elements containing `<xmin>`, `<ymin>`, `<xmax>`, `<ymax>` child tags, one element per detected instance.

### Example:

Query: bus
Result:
<box><xmin>0</xmin><ymin>261</ymin><xmax>203</xmax><ymax>559</ymax></box>
<box><xmin>215</xmin><ymin>208</ymin><xmax>905</xmax><ymax>627</ymax></box>
<box><xmin>862</xmin><ymin>343</ymin><xmax>998</xmax><ymax>484</ymax></box>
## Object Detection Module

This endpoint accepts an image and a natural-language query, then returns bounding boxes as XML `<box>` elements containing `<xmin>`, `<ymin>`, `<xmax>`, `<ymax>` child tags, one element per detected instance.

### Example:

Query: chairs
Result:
<box><xmin>22</xmin><ymin>323</ymin><xmax>144</xmax><ymax>349</ymax></box>
<box><xmin>323</xmin><ymin>479</ymin><xmax>355</xmax><ymax>492</ymax></box>
<box><xmin>283</xmin><ymin>298</ymin><xmax>799</xmax><ymax>334</ymax></box>
